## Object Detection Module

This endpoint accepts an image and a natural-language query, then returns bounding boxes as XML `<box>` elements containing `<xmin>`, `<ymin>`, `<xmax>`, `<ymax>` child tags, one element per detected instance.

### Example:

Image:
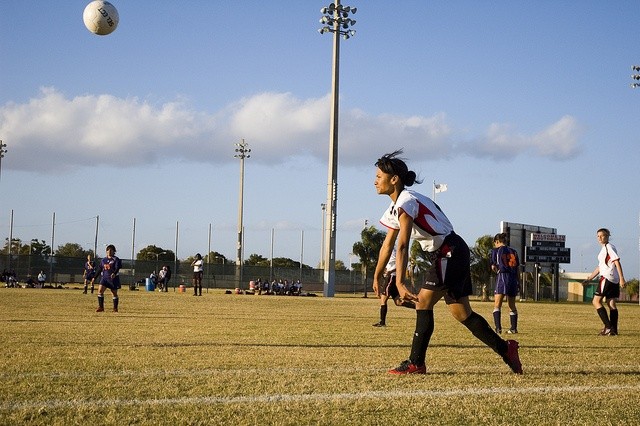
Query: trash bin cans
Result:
<box><xmin>146</xmin><ymin>278</ymin><xmax>156</xmax><ymax>291</ymax></box>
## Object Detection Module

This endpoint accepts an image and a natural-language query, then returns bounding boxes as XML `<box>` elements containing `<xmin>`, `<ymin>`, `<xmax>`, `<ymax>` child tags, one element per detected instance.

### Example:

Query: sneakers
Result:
<box><xmin>83</xmin><ymin>291</ymin><xmax>87</xmax><ymax>294</ymax></box>
<box><xmin>492</xmin><ymin>328</ymin><xmax>502</xmax><ymax>335</ymax></box>
<box><xmin>505</xmin><ymin>328</ymin><xmax>518</xmax><ymax>334</ymax></box>
<box><xmin>604</xmin><ymin>332</ymin><xmax>616</xmax><ymax>337</ymax></box>
<box><xmin>112</xmin><ymin>308</ymin><xmax>118</xmax><ymax>312</ymax></box>
<box><xmin>598</xmin><ymin>327</ymin><xmax>610</xmax><ymax>335</ymax></box>
<box><xmin>387</xmin><ymin>359</ymin><xmax>426</xmax><ymax>375</ymax></box>
<box><xmin>96</xmin><ymin>306</ymin><xmax>104</xmax><ymax>312</ymax></box>
<box><xmin>372</xmin><ymin>322</ymin><xmax>386</xmax><ymax>327</ymax></box>
<box><xmin>503</xmin><ymin>340</ymin><xmax>523</xmax><ymax>375</ymax></box>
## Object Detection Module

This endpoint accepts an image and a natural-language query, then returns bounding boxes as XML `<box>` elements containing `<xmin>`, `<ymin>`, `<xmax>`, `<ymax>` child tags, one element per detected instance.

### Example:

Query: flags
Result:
<box><xmin>435</xmin><ymin>184</ymin><xmax>447</xmax><ymax>193</ymax></box>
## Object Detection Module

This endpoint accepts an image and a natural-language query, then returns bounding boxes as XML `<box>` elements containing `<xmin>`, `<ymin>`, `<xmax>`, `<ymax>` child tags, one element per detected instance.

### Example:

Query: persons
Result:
<box><xmin>372</xmin><ymin>150</ymin><xmax>523</xmax><ymax>376</ymax></box>
<box><xmin>158</xmin><ymin>267</ymin><xmax>167</xmax><ymax>292</ymax></box>
<box><xmin>161</xmin><ymin>266</ymin><xmax>171</xmax><ymax>292</ymax></box>
<box><xmin>490</xmin><ymin>233</ymin><xmax>521</xmax><ymax>334</ymax></box>
<box><xmin>295</xmin><ymin>279</ymin><xmax>302</xmax><ymax>294</ymax></box>
<box><xmin>582</xmin><ymin>229</ymin><xmax>627</xmax><ymax>336</ymax></box>
<box><xmin>83</xmin><ymin>254</ymin><xmax>97</xmax><ymax>294</ymax></box>
<box><xmin>2</xmin><ymin>269</ymin><xmax>9</xmax><ymax>286</ymax></box>
<box><xmin>92</xmin><ymin>245</ymin><xmax>121</xmax><ymax>312</ymax></box>
<box><xmin>373</xmin><ymin>239</ymin><xmax>416</xmax><ymax>328</ymax></box>
<box><xmin>255</xmin><ymin>278</ymin><xmax>262</xmax><ymax>295</ymax></box>
<box><xmin>150</xmin><ymin>270</ymin><xmax>158</xmax><ymax>289</ymax></box>
<box><xmin>38</xmin><ymin>270</ymin><xmax>46</xmax><ymax>288</ymax></box>
<box><xmin>283</xmin><ymin>280</ymin><xmax>289</xmax><ymax>295</ymax></box>
<box><xmin>289</xmin><ymin>281</ymin><xmax>296</xmax><ymax>296</ymax></box>
<box><xmin>191</xmin><ymin>253</ymin><xmax>204</xmax><ymax>296</ymax></box>
<box><xmin>263</xmin><ymin>280</ymin><xmax>270</xmax><ymax>294</ymax></box>
<box><xmin>8</xmin><ymin>270</ymin><xmax>17</xmax><ymax>287</ymax></box>
<box><xmin>278</xmin><ymin>279</ymin><xmax>284</xmax><ymax>295</ymax></box>
<box><xmin>272</xmin><ymin>279</ymin><xmax>280</xmax><ymax>295</ymax></box>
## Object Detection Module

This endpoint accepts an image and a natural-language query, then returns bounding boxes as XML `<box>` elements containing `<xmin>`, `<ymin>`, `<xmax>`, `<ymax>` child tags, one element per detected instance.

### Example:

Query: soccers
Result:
<box><xmin>82</xmin><ymin>0</ymin><xmax>118</xmax><ymax>36</ymax></box>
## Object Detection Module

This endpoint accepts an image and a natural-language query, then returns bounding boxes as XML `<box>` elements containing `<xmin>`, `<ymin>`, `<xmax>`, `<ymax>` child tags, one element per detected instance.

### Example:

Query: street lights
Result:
<box><xmin>235</xmin><ymin>139</ymin><xmax>251</xmax><ymax>292</ymax></box>
<box><xmin>319</xmin><ymin>2</ymin><xmax>357</xmax><ymax>297</ymax></box>
